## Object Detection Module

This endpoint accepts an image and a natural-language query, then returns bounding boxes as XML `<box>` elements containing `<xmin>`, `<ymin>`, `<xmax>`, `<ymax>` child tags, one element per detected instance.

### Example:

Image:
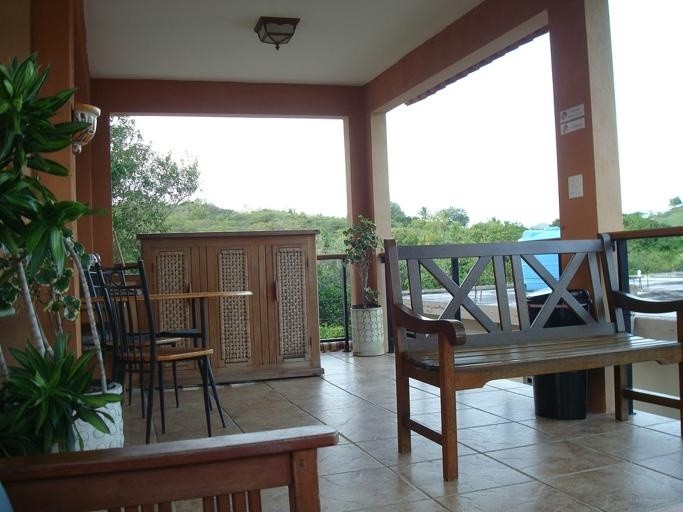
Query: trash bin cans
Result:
<box><xmin>527</xmin><ymin>290</ymin><xmax>592</xmax><ymax>420</ymax></box>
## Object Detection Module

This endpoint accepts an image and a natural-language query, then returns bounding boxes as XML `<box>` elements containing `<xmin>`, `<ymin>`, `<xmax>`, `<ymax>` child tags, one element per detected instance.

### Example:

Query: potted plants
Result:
<box><xmin>0</xmin><ymin>50</ymin><xmax>125</xmax><ymax>458</ymax></box>
<box><xmin>340</xmin><ymin>213</ymin><xmax>385</xmax><ymax>359</ymax></box>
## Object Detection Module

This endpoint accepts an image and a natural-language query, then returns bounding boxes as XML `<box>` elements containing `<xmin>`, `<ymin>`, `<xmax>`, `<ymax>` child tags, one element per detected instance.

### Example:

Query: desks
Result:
<box><xmin>77</xmin><ymin>288</ymin><xmax>254</xmax><ymax>438</ymax></box>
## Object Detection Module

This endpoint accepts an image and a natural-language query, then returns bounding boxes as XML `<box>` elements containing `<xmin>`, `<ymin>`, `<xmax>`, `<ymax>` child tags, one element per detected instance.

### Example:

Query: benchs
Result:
<box><xmin>383</xmin><ymin>233</ymin><xmax>683</xmax><ymax>483</ymax></box>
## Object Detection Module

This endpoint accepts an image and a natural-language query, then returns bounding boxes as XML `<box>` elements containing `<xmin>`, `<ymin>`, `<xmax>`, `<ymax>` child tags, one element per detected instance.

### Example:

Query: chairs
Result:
<box><xmin>74</xmin><ymin>257</ymin><xmax>227</xmax><ymax>446</ymax></box>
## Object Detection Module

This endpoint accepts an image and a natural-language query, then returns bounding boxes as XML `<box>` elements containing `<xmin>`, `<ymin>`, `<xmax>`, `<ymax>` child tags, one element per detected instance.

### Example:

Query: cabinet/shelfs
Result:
<box><xmin>263</xmin><ymin>233</ymin><xmax>321</xmax><ymax>381</ymax></box>
<box><xmin>139</xmin><ymin>235</ymin><xmax>263</xmax><ymax>390</ymax></box>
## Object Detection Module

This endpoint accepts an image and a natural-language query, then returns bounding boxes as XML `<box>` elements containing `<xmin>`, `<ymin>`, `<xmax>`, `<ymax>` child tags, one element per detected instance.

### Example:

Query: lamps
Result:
<box><xmin>71</xmin><ymin>101</ymin><xmax>102</xmax><ymax>156</ymax></box>
<box><xmin>252</xmin><ymin>15</ymin><xmax>301</xmax><ymax>53</ymax></box>
